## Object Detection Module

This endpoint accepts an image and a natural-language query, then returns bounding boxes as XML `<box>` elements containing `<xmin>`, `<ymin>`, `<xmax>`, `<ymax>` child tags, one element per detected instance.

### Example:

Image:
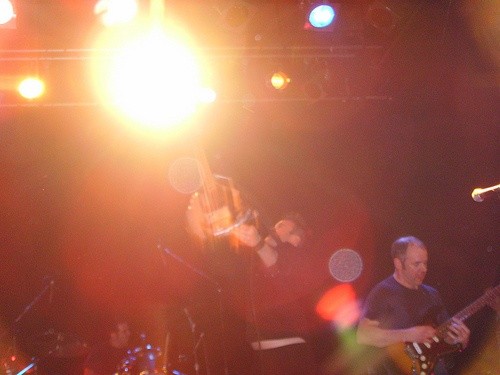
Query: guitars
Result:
<box><xmin>402</xmin><ymin>287</ymin><xmax>499</xmax><ymax>375</ymax></box>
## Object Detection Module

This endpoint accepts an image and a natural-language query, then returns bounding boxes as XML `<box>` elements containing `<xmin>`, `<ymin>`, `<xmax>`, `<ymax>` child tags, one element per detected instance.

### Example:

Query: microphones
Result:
<box><xmin>471</xmin><ymin>184</ymin><xmax>500</xmax><ymax>202</ymax></box>
